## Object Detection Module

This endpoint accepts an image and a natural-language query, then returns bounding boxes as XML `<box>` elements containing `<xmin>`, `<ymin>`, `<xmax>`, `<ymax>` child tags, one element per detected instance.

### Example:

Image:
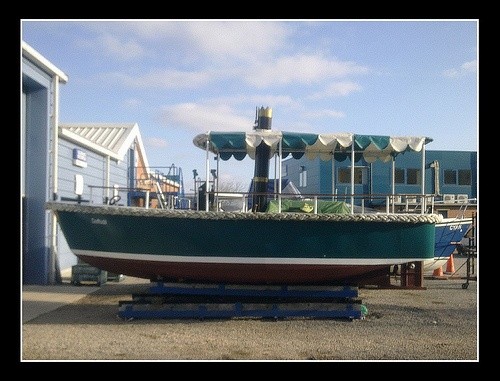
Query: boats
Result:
<box><xmin>46</xmin><ymin>105</ymin><xmax>444</xmax><ymax>286</ymax></box>
<box><xmin>388</xmin><ymin>217</ymin><xmax>473</xmax><ymax>277</ymax></box>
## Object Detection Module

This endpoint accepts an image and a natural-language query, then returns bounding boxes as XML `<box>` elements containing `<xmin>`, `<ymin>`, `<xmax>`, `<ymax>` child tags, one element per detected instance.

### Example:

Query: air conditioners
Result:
<box><xmin>405</xmin><ymin>194</ymin><xmax>416</xmax><ymax>203</ymax></box>
<box><xmin>443</xmin><ymin>194</ymin><xmax>456</xmax><ymax>204</ymax></box>
<box><xmin>457</xmin><ymin>194</ymin><xmax>469</xmax><ymax>203</ymax></box>
<box><xmin>390</xmin><ymin>195</ymin><xmax>401</xmax><ymax>203</ymax></box>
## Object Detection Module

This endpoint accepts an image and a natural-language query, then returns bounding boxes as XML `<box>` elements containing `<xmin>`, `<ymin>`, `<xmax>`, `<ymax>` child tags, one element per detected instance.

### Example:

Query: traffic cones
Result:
<box><xmin>429</xmin><ymin>265</ymin><xmax>449</xmax><ymax>280</ymax></box>
<box><xmin>443</xmin><ymin>254</ymin><xmax>459</xmax><ymax>275</ymax></box>
